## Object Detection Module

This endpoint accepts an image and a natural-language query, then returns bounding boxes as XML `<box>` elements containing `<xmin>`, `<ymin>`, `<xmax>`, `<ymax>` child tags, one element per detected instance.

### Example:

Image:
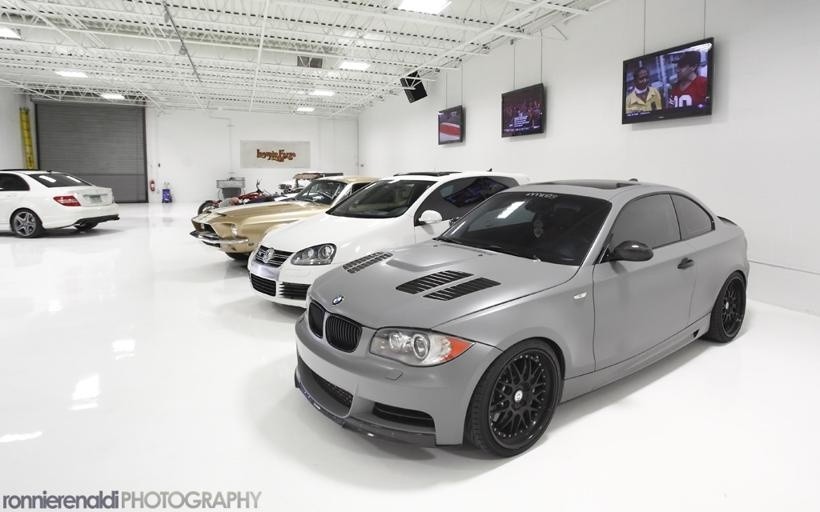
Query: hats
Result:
<box><xmin>672</xmin><ymin>51</ymin><xmax>701</xmax><ymax>68</ymax></box>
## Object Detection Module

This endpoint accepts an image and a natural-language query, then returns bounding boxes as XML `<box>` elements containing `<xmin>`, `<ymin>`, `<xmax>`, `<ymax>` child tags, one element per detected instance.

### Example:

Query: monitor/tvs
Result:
<box><xmin>501</xmin><ymin>83</ymin><xmax>545</xmax><ymax>137</ymax></box>
<box><xmin>438</xmin><ymin>105</ymin><xmax>463</xmax><ymax>145</ymax></box>
<box><xmin>622</xmin><ymin>37</ymin><xmax>714</xmax><ymax>124</ymax></box>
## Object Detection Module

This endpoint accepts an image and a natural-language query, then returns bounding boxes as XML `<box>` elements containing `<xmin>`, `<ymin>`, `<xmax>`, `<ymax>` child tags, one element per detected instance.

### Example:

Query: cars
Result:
<box><xmin>189</xmin><ymin>170</ymin><xmax>751</xmax><ymax>459</ymax></box>
<box><xmin>0</xmin><ymin>168</ymin><xmax>121</xmax><ymax>238</ymax></box>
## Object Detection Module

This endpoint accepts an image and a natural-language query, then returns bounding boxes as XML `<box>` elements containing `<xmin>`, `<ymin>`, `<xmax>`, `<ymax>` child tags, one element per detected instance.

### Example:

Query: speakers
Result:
<box><xmin>400</xmin><ymin>71</ymin><xmax>428</xmax><ymax>104</ymax></box>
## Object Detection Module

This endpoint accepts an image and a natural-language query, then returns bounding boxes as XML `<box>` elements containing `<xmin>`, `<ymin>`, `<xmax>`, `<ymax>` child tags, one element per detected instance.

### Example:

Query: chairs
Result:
<box><xmin>529</xmin><ymin>208</ymin><xmax>576</xmax><ymax>244</ymax></box>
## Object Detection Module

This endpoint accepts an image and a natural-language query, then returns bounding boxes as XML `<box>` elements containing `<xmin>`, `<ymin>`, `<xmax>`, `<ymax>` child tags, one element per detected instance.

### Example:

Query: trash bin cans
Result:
<box><xmin>163</xmin><ymin>189</ymin><xmax>171</xmax><ymax>202</ymax></box>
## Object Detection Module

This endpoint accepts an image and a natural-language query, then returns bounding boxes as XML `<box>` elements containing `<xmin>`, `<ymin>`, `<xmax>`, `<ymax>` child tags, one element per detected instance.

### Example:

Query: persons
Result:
<box><xmin>623</xmin><ymin>66</ymin><xmax>663</xmax><ymax>116</ymax></box>
<box><xmin>666</xmin><ymin>52</ymin><xmax>707</xmax><ymax>109</ymax></box>
<box><xmin>218</xmin><ymin>195</ymin><xmax>239</xmax><ymax>208</ymax></box>
<box><xmin>503</xmin><ymin>98</ymin><xmax>541</xmax><ymax>129</ymax></box>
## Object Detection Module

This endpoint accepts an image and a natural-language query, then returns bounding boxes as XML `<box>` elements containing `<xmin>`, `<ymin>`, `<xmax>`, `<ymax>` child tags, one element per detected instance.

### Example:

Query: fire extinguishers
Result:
<box><xmin>151</xmin><ymin>180</ymin><xmax>154</xmax><ymax>190</ymax></box>
